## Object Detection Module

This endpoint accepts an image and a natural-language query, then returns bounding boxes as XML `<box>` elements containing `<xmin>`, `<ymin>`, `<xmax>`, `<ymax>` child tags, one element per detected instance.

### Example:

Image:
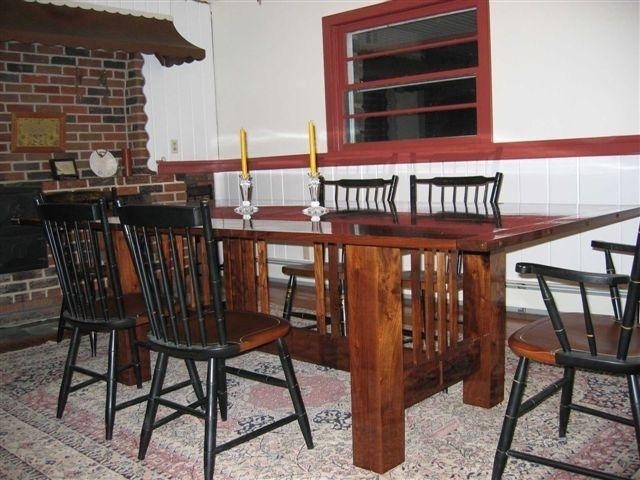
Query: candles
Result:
<box><xmin>309</xmin><ymin>121</ymin><xmax>317</xmax><ymax>178</ymax></box>
<box><xmin>240</xmin><ymin>129</ymin><xmax>248</xmax><ymax>177</ymax></box>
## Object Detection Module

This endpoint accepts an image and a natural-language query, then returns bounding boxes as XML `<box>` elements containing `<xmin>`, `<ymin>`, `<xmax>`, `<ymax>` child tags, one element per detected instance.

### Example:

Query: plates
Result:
<box><xmin>88</xmin><ymin>149</ymin><xmax>118</xmax><ymax>180</ymax></box>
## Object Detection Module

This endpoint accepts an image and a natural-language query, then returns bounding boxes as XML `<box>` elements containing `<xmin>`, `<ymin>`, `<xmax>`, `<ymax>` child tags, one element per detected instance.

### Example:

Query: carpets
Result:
<box><xmin>1</xmin><ymin>300</ymin><xmax>640</xmax><ymax>480</ymax></box>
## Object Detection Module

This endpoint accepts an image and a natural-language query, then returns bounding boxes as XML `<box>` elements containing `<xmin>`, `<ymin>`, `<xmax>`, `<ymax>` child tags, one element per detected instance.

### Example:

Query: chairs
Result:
<box><xmin>28</xmin><ymin>186</ymin><xmax>203</xmax><ymax>441</ymax></box>
<box><xmin>111</xmin><ymin>184</ymin><xmax>316</xmax><ymax>479</ymax></box>
<box><xmin>491</xmin><ymin>231</ymin><xmax>639</xmax><ymax>478</ymax></box>
<box><xmin>281</xmin><ymin>175</ymin><xmax>399</xmax><ymax>330</ymax></box>
<box><xmin>398</xmin><ymin>172</ymin><xmax>507</xmax><ymax>395</ymax></box>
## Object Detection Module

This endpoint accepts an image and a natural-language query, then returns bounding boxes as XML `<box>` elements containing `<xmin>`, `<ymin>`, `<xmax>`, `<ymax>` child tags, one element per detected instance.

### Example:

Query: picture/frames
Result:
<box><xmin>9</xmin><ymin>112</ymin><xmax>66</xmax><ymax>151</ymax></box>
<box><xmin>49</xmin><ymin>158</ymin><xmax>79</xmax><ymax>178</ymax></box>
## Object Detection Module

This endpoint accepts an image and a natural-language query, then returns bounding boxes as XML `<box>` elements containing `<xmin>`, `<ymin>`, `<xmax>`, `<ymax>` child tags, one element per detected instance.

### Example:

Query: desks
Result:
<box><xmin>85</xmin><ymin>201</ymin><xmax>639</xmax><ymax>474</ymax></box>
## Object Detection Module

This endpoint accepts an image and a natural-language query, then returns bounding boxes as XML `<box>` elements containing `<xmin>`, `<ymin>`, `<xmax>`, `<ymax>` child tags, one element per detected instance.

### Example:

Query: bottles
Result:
<box><xmin>123</xmin><ymin>148</ymin><xmax>133</xmax><ymax>177</ymax></box>
